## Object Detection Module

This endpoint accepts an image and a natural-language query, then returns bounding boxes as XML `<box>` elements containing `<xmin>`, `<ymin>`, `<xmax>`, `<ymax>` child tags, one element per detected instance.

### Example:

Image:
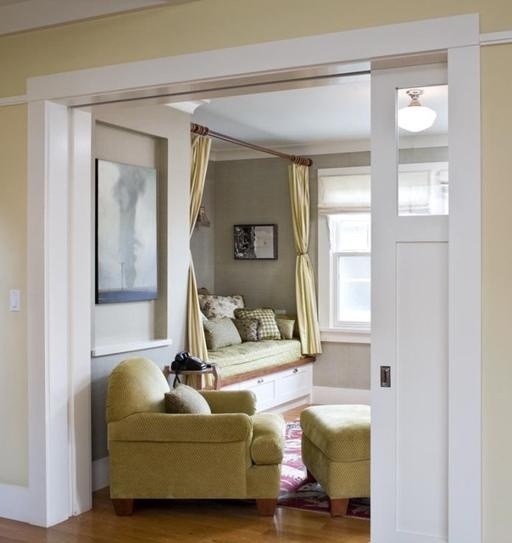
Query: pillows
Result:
<box><xmin>197</xmin><ymin>295</ymin><xmax>245</xmax><ymax>320</ymax></box>
<box><xmin>276</xmin><ymin>319</ymin><xmax>295</xmax><ymax>340</ymax></box>
<box><xmin>234</xmin><ymin>308</ymin><xmax>281</xmax><ymax>339</ymax></box>
<box><xmin>204</xmin><ymin>316</ymin><xmax>241</xmax><ymax>352</ymax></box>
<box><xmin>165</xmin><ymin>383</ymin><xmax>212</xmax><ymax>414</ymax></box>
<box><xmin>234</xmin><ymin>318</ymin><xmax>260</xmax><ymax>342</ymax></box>
<box><xmin>275</xmin><ymin>314</ymin><xmax>298</xmax><ymax>336</ymax></box>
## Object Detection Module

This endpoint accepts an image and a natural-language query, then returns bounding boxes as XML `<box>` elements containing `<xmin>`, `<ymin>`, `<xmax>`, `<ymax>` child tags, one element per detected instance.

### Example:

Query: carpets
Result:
<box><xmin>278</xmin><ymin>417</ymin><xmax>370</xmax><ymax>521</ymax></box>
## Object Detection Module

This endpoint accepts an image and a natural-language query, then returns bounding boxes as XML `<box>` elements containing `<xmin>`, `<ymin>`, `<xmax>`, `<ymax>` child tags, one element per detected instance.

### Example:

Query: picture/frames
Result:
<box><xmin>233</xmin><ymin>224</ymin><xmax>279</xmax><ymax>261</ymax></box>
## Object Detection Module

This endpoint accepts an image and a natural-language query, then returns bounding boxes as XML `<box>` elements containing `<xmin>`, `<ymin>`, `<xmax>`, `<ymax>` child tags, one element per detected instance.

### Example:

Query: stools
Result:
<box><xmin>300</xmin><ymin>404</ymin><xmax>371</xmax><ymax>516</ymax></box>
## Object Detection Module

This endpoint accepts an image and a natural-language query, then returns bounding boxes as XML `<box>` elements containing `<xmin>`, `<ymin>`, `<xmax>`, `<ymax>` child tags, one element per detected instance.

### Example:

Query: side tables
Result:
<box><xmin>167</xmin><ymin>364</ymin><xmax>218</xmax><ymax>391</ymax></box>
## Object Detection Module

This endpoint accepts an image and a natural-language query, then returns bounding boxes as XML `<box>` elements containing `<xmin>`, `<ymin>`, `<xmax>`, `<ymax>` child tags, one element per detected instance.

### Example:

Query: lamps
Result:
<box><xmin>396</xmin><ymin>87</ymin><xmax>437</xmax><ymax>134</ymax></box>
<box><xmin>194</xmin><ymin>206</ymin><xmax>210</xmax><ymax>231</ymax></box>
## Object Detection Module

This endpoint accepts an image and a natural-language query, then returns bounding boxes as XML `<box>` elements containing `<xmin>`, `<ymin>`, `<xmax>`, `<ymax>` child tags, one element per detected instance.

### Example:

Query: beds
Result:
<box><xmin>206</xmin><ymin>339</ymin><xmax>313</xmax><ymax>414</ymax></box>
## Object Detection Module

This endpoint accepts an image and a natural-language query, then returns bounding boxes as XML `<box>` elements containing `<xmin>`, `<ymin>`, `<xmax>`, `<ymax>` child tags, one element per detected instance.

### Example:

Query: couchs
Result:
<box><xmin>106</xmin><ymin>357</ymin><xmax>282</xmax><ymax>517</ymax></box>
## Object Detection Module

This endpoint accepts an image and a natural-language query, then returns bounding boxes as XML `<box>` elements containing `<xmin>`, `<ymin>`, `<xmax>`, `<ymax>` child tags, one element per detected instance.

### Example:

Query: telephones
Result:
<box><xmin>171</xmin><ymin>350</ymin><xmax>207</xmax><ymax>372</ymax></box>
